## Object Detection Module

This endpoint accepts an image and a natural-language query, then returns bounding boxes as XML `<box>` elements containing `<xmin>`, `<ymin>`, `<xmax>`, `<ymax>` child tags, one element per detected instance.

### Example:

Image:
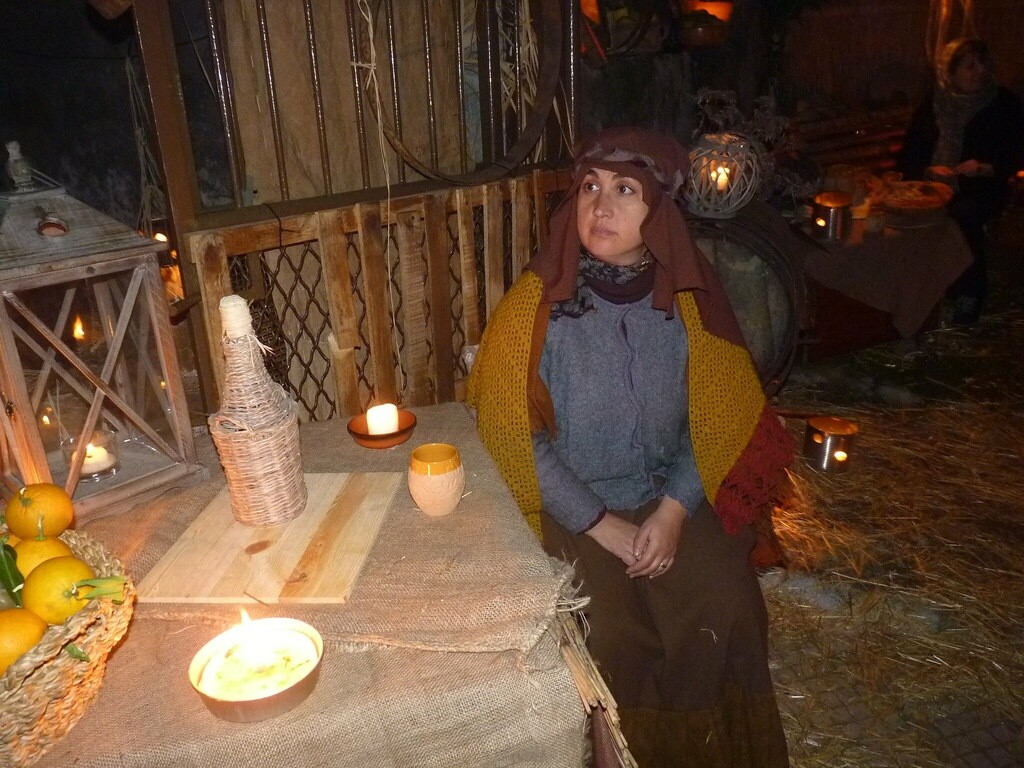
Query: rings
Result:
<box><xmin>659</xmin><ymin>562</ymin><xmax>666</xmax><ymax>569</ymax></box>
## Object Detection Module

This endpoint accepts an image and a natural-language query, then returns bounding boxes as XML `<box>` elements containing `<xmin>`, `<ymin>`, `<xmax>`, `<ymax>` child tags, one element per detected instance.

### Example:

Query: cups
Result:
<box><xmin>60</xmin><ymin>430</ymin><xmax>121</xmax><ymax>483</ymax></box>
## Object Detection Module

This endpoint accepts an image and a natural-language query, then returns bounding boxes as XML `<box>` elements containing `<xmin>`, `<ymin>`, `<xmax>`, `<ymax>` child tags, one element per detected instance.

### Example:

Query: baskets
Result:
<box><xmin>0</xmin><ymin>521</ymin><xmax>135</xmax><ymax>768</ymax></box>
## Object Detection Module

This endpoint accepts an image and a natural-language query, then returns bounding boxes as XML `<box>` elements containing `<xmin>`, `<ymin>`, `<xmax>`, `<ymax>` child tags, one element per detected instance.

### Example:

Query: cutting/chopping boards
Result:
<box><xmin>130</xmin><ymin>472</ymin><xmax>402</xmax><ymax>605</ymax></box>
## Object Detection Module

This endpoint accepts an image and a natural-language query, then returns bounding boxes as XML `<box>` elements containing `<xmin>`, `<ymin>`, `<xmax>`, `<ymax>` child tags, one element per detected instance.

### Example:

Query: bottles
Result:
<box><xmin>408</xmin><ymin>443</ymin><xmax>466</xmax><ymax>517</ymax></box>
<box><xmin>208</xmin><ymin>295</ymin><xmax>308</xmax><ymax>528</ymax></box>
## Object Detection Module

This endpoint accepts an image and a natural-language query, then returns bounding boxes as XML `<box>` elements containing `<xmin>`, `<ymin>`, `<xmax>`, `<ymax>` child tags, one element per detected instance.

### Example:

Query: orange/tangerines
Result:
<box><xmin>0</xmin><ymin>484</ymin><xmax>97</xmax><ymax>680</ymax></box>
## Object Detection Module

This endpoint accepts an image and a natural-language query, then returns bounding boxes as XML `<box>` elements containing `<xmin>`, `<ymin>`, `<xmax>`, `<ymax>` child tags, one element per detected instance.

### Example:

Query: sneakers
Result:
<box><xmin>952</xmin><ymin>295</ymin><xmax>983</xmax><ymax>325</ymax></box>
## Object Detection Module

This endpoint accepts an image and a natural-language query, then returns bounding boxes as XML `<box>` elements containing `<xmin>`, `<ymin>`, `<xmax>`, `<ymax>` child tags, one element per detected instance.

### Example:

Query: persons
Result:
<box><xmin>464</xmin><ymin>129</ymin><xmax>795</xmax><ymax>768</ymax></box>
<box><xmin>898</xmin><ymin>36</ymin><xmax>1024</xmax><ymax>327</ymax></box>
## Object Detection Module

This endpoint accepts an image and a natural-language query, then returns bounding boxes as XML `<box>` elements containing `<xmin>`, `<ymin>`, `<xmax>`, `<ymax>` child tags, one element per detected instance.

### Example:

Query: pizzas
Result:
<box><xmin>877</xmin><ymin>180</ymin><xmax>954</xmax><ymax>208</ymax></box>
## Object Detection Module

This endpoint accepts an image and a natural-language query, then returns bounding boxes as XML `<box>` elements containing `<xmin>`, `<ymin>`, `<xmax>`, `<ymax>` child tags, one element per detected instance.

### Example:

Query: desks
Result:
<box><xmin>790</xmin><ymin>215</ymin><xmax>973</xmax><ymax>362</ymax></box>
<box><xmin>38</xmin><ymin>402</ymin><xmax>640</xmax><ymax>768</ymax></box>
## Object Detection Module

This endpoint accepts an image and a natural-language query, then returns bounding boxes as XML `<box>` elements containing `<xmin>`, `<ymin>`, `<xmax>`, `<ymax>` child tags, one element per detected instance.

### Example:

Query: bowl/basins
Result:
<box><xmin>187</xmin><ymin>618</ymin><xmax>323</xmax><ymax>725</ymax></box>
<box><xmin>347</xmin><ymin>408</ymin><xmax>415</xmax><ymax>449</ymax></box>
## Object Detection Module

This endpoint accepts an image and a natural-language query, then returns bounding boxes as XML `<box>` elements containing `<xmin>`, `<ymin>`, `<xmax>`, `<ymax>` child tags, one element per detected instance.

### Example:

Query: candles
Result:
<box><xmin>71</xmin><ymin>444</ymin><xmax>108</xmax><ymax>470</ymax></box>
<box><xmin>367</xmin><ymin>402</ymin><xmax>399</xmax><ymax>435</ymax></box>
